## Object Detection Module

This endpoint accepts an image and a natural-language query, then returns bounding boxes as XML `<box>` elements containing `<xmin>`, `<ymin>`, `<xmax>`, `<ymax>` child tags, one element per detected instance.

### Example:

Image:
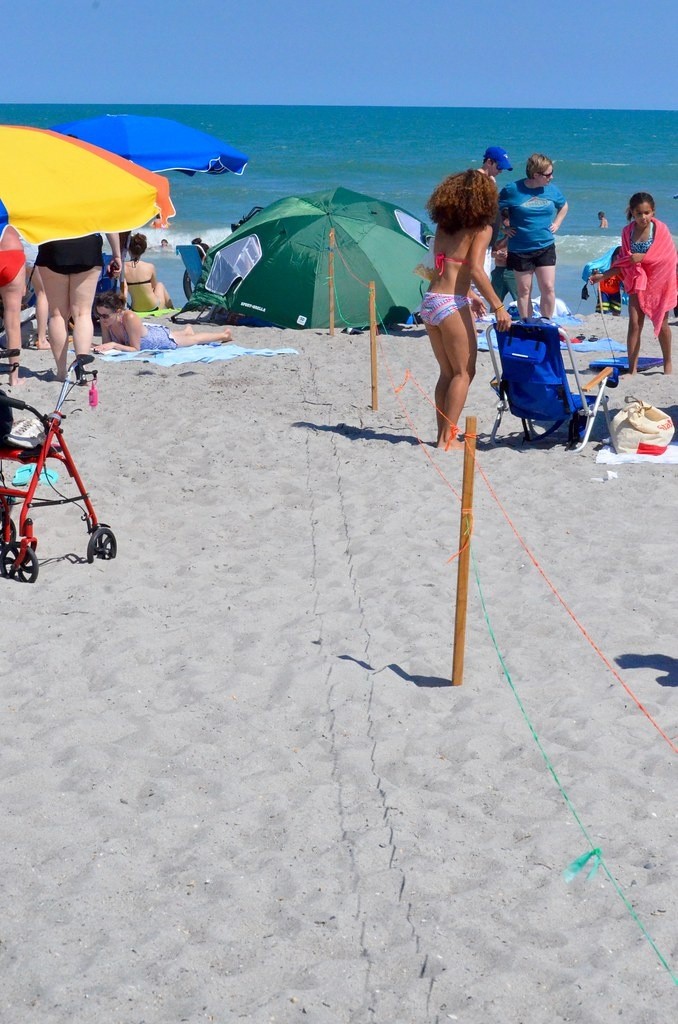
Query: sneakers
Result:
<box><xmin>7</xmin><ymin>417</ymin><xmax>46</xmax><ymax>450</ymax></box>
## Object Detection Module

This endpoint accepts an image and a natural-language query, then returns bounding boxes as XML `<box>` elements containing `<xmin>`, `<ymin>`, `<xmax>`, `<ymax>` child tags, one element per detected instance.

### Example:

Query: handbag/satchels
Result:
<box><xmin>609</xmin><ymin>395</ymin><xmax>675</xmax><ymax>455</ymax></box>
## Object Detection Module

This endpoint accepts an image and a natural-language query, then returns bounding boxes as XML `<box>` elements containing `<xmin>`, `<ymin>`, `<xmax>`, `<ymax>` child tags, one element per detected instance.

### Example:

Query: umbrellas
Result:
<box><xmin>0</xmin><ymin>125</ymin><xmax>176</xmax><ymax>245</ymax></box>
<box><xmin>49</xmin><ymin>114</ymin><xmax>249</xmax><ymax>175</ymax></box>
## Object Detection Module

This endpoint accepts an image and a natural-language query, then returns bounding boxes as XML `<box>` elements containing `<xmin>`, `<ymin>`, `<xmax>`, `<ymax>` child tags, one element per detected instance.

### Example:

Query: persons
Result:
<box><xmin>0</xmin><ymin>225</ymin><xmax>26</xmax><ymax>387</ymax></box>
<box><xmin>93</xmin><ymin>292</ymin><xmax>235</xmax><ymax>353</ymax></box>
<box><xmin>594</xmin><ymin>246</ymin><xmax>622</xmax><ymax>316</ymax></box>
<box><xmin>161</xmin><ymin>239</ymin><xmax>168</xmax><ymax>247</ymax></box>
<box><xmin>35</xmin><ymin>232</ymin><xmax>122</xmax><ymax>382</ymax></box>
<box><xmin>598</xmin><ymin>212</ymin><xmax>608</xmax><ymax>228</ymax></box>
<box><xmin>118</xmin><ymin>231</ymin><xmax>174</xmax><ymax>312</ymax></box>
<box><xmin>191</xmin><ymin>238</ymin><xmax>211</xmax><ymax>261</ymax></box>
<box><xmin>19</xmin><ymin>235</ymin><xmax>50</xmax><ymax>349</ymax></box>
<box><xmin>420</xmin><ymin>171</ymin><xmax>512</xmax><ymax>449</ymax></box>
<box><xmin>478</xmin><ymin>146</ymin><xmax>568</xmax><ymax>319</ymax></box>
<box><xmin>150</xmin><ymin>214</ymin><xmax>163</xmax><ymax>228</ymax></box>
<box><xmin>589</xmin><ymin>193</ymin><xmax>678</xmax><ymax>375</ymax></box>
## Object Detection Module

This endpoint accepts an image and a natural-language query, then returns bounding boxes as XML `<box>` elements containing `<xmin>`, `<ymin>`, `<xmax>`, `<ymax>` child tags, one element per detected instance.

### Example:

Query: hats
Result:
<box><xmin>484</xmin><ymin>146</ymin><xmax>513</xmax><ymax>171</ymax></box>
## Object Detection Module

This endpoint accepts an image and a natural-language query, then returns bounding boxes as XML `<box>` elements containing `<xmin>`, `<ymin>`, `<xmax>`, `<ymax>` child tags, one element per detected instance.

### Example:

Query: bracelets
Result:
<box><xmin>495</xmin><ymin>305</ymin><xmax>504</xmax><ymax>312</ymax></box>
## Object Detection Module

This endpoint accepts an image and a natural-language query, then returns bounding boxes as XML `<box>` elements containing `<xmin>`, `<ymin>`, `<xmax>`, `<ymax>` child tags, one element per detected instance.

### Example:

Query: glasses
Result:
<box><xmin>537</xmin><ymin>169</ymin><xmax>553</xmax><ymax>178</ymax></box>
<box><xmin>97</xmin><ymin>310</ymin><xmax>114</xmax><ymax>319</ymax></box>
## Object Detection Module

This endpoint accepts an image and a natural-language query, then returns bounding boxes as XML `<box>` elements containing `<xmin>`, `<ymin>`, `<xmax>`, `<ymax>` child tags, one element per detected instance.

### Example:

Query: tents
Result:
<box><xmin>172</xmin><ymin>186</ymin><xmax>435</xmax><ymax>330</ymax></box>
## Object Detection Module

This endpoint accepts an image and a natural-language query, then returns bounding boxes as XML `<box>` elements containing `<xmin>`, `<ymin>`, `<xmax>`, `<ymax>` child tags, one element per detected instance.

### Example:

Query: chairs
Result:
<box><xmin>485</xmin><ymin>317</ymin><xmax>615</xmax><ymax>451</ymax></box>
<box><xmin>176</xmin><ymin>244</ymin><xmax>207</xmax><ymax>285</ymax></box>
